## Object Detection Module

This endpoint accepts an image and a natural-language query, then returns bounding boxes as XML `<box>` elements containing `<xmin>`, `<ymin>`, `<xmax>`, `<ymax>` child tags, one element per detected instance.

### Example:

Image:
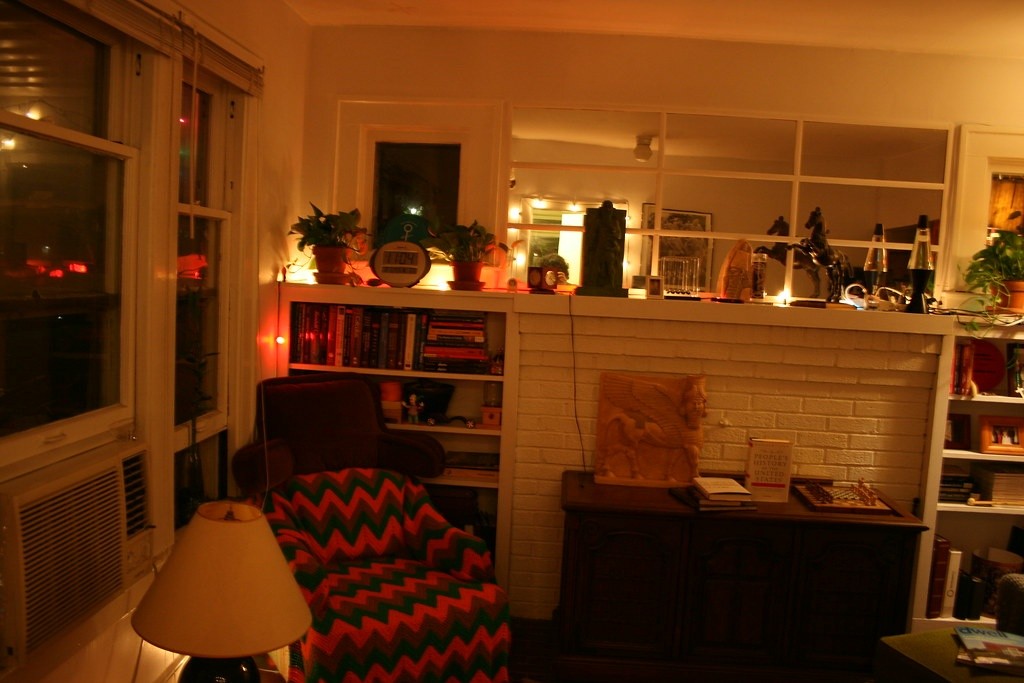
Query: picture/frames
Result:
<box><xmin>640</xmin><ymin>203</ymin><xmax>713</xmax><ymax>293</ymax></box>
<box><xmin>978</xmin><ymin>414</ymin><xmax>1024</xmax><ymax>456</ymax></box>
<box><xmin>944</xmin><ymin>413</ymin><xmax>971</xmax><ymax>449</ymax></box>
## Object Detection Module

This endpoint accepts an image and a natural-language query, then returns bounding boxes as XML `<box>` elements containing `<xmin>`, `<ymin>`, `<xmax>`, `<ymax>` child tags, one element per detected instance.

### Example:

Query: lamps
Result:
<box><xmin>419</xmin><ymin>220</ymin><xmax>508</xmax><ymax>292</ymax></box>
<box><xmin>862</xmin><ymin>224</ymin><xmax>888</xmax><ymax>299</ymax></box>
<box><xmin>906</xmin><ymin>214</ymin><xmax>934</xmax><ymax>314</ymax></box>
<box><xmin>131</xmin><ymin>501</ymin><xmax>313</xmax><ymax>683</ymax></box>
<box><xmin>632</xmin><ymin>134</ymin><xmax>653</xmax><ymax>163</ymax></box>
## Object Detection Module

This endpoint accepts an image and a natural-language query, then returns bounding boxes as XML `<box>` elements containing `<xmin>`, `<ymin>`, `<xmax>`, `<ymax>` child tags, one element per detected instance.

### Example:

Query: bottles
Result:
<box><xmin>908</xmin><ymin>215</ymin><xmax>935</xmax><ymax>270</ymax></box>
<box><xmin>864</xmin><ymin>223</ymin><xmax>888</xmax><ymax>272</ymax></box>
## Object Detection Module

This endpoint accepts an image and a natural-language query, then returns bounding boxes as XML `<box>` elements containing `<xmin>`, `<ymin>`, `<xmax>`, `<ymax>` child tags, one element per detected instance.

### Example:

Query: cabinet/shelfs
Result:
<box><xmin>548</xmin><ymin>470</ymin><xmax>929</xmax><ymax>683</ymax></box>
<box><xmin>904</xmin><ymin>316</ymin><xmax>1024</xmax><ymax>633</ymax></box>
<box><xmin>277</xmin><ymin>282</ymin><xmax>520</xmax><ymax>591</ymax></box>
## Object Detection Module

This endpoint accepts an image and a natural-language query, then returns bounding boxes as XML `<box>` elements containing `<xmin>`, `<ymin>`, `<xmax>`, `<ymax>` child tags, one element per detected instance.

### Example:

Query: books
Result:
<box><xmin>925</xmin><ymin>533</ymin><xmax>1024</xmax><ymax>677</ymax></box>
<box><xmin>744</xmin><ymin>436</ymin><xmax>792</xmax><ymax>503</ymax></box>
<box><xmin>938</xmin><ymin>464</ymin><xmax>1024</xmax><ymax>509</ymax></box>
<box><xmin>669</xmin><ymin>476</ymin><xmax>759</xmax><ymax>512</ymax></box>
<box><xmin>952</xmin><ymin>342</ymin><xmax>976</xmax><ymax>395</ymax></box>
<box><xmin>287</xmin><ymin>302</ymin><xmax>504</xmax><ymax>375</ymax></box>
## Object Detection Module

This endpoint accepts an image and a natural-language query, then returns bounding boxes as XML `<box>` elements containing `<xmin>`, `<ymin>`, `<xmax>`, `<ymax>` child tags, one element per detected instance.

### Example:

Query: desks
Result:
<box><xmin>873</xmin><ymin>626</ymin><xmax>1024</xmax><ymax>683</ymax></box>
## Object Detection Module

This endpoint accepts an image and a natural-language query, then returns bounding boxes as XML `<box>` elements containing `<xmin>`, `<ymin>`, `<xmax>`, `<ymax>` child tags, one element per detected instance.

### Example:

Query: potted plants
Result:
<box><xmin>956</xmin><ymin>211</ymin><xmax>1024</xmax><ymax>340</ymax></box>
<box><xmin>527</xmin><ymin>254</ymin><xmax>570</xmax><ymax>295</ymax></box>
<box><xmin>288</xmin><ymin>201</ymin><xmax>373</xmax><ymax>285</ymax></box>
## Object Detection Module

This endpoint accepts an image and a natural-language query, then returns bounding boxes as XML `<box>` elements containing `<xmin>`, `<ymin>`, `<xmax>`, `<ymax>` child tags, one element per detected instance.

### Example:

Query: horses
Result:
<box><xmin>753</xmin><ymin>214</ymin><xmax>819</xmax><ymax>298</ymax></box>
<box><xmin>786</xmin><ymin>207</ymin><xmax>847</xmax><ymax>302</ymax></box>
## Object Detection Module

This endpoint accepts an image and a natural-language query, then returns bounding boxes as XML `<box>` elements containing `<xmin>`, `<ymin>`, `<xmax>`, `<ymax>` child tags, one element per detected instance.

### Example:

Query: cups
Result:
<box><xmin>971</xmin><ymin>548</ymin><xmax>1024</xmax><ymax>614</ymax></box>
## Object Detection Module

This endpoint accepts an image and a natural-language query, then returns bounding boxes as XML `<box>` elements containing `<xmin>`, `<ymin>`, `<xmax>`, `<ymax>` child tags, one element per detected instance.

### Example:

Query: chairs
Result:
<box><xmin>229</xmin><ymin>371</ymin><xmax>513</xmax><ymax>683</ymax></box>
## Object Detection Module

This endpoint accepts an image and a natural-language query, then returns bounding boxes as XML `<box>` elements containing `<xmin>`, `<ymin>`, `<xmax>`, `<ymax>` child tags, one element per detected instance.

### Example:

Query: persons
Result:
<box><xmin>402</xmin><ymin>393</ymin><xmax>423</xmax><ymax>425</ymax></box>
<box><xmin>1002</xmin><ymin>429</ymin><xmax>1013</xmax><ymax>445</ymax></box>
